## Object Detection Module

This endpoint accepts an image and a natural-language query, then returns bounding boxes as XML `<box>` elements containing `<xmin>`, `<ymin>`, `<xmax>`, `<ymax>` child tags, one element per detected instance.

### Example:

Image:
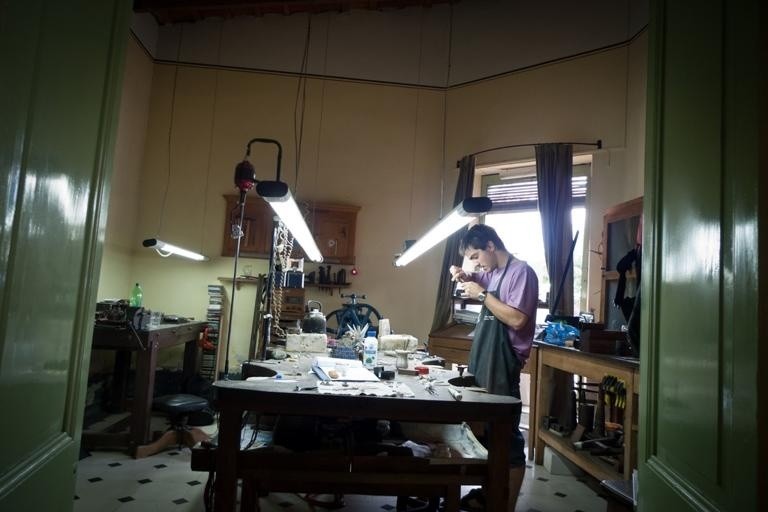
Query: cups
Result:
<box><xmin>149</xmin><ymin>310</ymin><xmax>164</xmax><ymax>326</ymax></box>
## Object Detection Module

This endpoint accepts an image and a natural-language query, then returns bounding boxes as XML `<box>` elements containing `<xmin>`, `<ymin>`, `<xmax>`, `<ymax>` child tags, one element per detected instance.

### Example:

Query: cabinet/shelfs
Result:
<box><xmin>599</xmin><ymin>195</ymin><xmax>643</xmax><ymax>333</ymax></box>
<box><xmin>220</xmin><ymin>193</ymin><xmax>362</xmax><ymax>265</ymax></box>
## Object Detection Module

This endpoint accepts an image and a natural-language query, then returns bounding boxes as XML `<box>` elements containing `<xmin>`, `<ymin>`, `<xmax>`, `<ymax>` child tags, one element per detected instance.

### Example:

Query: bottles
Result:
<box><xmin>396</xmin><ymin>352</ymin><xmax>408</xmax><ymax>369</ymax></box>
<box><xmin>362</xmin><ymin>331</ymin><xmax>379</xmax><ymax>368</ymax></box>
<box><xmin>131</xmin><ymin>283</ymin><xmax>144</xmax><ymax>307</ymax></box>
<box><xmin>336</xmin><ymin>268</ymin><xmax>347</xmax><ymax>284</ymax></box>
<box><xmin>378</xmin><ymin>420</ymin><xmax>391</xmax><ymax>441</ymax></box>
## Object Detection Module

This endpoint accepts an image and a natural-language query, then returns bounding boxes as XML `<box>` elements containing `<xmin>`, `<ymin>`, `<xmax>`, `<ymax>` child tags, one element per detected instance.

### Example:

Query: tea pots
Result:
<box><xmin>303</xmin><ymin>299</ymin><xmax>327</xmax><ymax>333</ymax></box>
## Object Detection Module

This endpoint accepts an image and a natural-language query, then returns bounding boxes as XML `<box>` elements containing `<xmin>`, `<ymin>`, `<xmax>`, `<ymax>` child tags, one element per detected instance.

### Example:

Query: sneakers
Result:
<box><xmin>460</xmin><ymin>488</ymin><xmax>486</xmax><ymax>510</ymax></box>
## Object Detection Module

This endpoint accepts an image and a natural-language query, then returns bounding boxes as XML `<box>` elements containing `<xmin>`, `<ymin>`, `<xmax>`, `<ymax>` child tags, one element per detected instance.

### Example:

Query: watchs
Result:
<box><xmin>477</xmin><ymin>290</ymin><xmax>488</xmax><ymax>303</ymax></box>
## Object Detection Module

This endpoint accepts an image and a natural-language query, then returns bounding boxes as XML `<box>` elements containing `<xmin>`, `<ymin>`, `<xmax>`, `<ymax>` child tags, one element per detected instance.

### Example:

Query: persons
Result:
<box><xmin>447</xmin><ymin>223</ymin><xmax>542</xmax><ymax>512</ymax></box>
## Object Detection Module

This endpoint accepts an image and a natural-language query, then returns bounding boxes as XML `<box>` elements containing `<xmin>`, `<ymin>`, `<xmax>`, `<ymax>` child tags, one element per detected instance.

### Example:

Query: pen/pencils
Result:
<box><xmin>347</xmin><ymin>323</ymin><xmax>369</xmax><ymax>352</ymax></box>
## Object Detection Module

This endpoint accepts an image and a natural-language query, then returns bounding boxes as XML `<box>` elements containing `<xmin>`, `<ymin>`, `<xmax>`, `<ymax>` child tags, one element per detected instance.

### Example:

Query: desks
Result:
<box><xmin>531</xmin><ymin>335</ymin><xmax>641</xmax><ymax>483</ymax></box>
<box><xmin>191</xmin><ymin>346</ymin><xmax>522</xmax><ymax>512</ymax></box>
<box><xmin>427</xmin><ymin>319</ymin><xmax>539</xmax><ymax>460</ymax></box>
<box><xmin>82</xmin><ymin>320</ymin><xmax>209</xmax><ymax>459</ymax></box>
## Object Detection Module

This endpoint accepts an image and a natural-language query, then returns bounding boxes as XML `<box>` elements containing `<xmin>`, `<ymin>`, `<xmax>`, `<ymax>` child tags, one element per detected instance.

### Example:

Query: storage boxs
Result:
<box><xmin>543</xmin><ymin>444</ymin><xmax>585</xmax><ymax>477</ymax></box>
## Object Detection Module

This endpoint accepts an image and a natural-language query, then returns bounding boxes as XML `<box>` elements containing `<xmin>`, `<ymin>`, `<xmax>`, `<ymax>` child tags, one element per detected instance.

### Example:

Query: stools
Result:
<box><xmin>153</xmin><ymin>393</ymin><xmax>209</xmax><ymax>454</ymax></box>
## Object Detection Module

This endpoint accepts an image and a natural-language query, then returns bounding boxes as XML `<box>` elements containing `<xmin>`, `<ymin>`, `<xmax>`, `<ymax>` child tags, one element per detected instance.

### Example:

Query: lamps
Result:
<box><xmin>255</xmin><ymin>9</ymin><xmax>330</xmax><ymax>264</ymax></box>
<box><xmin>390</xmin><ymin>2</ymin><xmax>493</xmax><ymax>269</ymax></box>
<box><xmin>142</xmin><ymin>15</ymin><xmax>223</xmax><ymax>264</ymax></box>
